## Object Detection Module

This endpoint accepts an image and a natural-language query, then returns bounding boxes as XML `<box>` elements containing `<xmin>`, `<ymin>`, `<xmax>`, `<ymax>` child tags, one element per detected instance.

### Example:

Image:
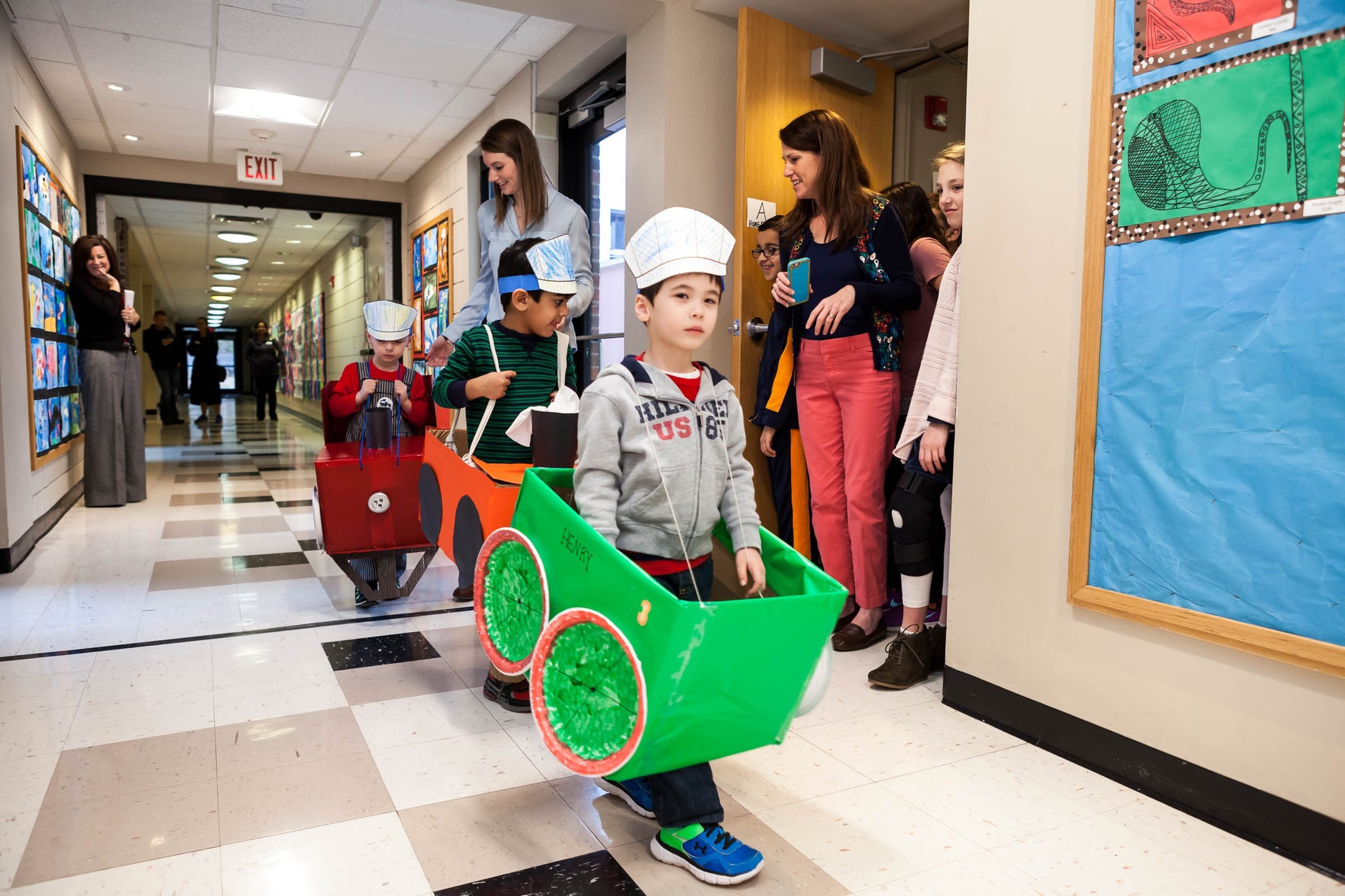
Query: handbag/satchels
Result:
<box><xmin>217</xmin><ymin>365</ymin><xmax>226</xmax><ymax>382</ymax></box>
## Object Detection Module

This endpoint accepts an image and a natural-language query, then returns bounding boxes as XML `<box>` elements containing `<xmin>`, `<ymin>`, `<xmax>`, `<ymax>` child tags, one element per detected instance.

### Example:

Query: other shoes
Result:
<box><xmin>162</xmin><ymin>419</ymin><xmax>184</xmax><ymax>425</ymax></box>
<box><xmin>195</xmin><ymin>415</ymin><xmax>207</xmax><ymax>423</ymax></box>
<box><xmin>832</xmin><ymin>611</ymin><xmax>888</xmax><ymax>652</ymax></box>
<box><xmin>453</xmin><ymin>585</ymin><xmax>473</xmax><ymax>600</ymax></box>
<box><xmin>215</xmin><ymin>415</ymin><xmax>222</xmax><ymax>423</ymax></box>
<box><xmin>923</xmin><ymin>623</ymin><xmax>946</xmax><ymax>674</ymax></box>
<box><xmin>258</xmin><ymin>417</ymin><xmax>264</xmax><ymax>420</ymax></box>
<box><xmin>831</xmin><ymin>602</ymin><xmax>860</xmax><ymax>634</ymax></box>
<box><xmin>868</xmin><ymin>624</ymin><xmax>928</xmax><ymax>689</ymax></box>
<box><xmin>271</xmin><ymin>417</ymin><xmax>278</xmax><ymax>420</ymax></box>
<box><xmin>354</xmin><ymin>580</ymin><xmax>379</xmax><ymax>607</ymax></box>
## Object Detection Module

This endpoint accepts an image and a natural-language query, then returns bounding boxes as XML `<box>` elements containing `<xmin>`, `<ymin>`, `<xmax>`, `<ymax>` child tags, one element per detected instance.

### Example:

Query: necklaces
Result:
<box><xmin>515</xmin><ymin>210</ymin><xmax>525</xmax><ymax>218</ymax></box>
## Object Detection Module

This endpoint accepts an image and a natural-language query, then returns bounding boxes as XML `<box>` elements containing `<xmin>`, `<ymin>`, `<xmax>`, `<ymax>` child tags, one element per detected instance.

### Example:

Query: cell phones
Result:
<box><xmin>786</xmin><ymin>255</ymin><xmax>811</xmax><ymax>308</ymax></box>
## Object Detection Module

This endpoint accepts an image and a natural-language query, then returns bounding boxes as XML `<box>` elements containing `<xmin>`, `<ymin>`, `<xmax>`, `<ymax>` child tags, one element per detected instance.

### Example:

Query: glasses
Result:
<box><xmin>751</xmin><ymin>247</ymin><xmax>780</xmax><ymax>258</ymax></box>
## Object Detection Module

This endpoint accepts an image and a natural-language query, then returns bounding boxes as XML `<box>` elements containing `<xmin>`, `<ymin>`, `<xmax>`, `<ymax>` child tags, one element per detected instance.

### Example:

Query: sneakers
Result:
<box><xmin>483</xmin><ymin>668</ymin><xmax>531</xmax><ymax>712</ymax></box>
<box><xmin>650</xmin><ymin>821</ymin><xmax>765</xmax><ymax>885</ymax></box>
<box><xmin>593</xmin><ymin>775</ymin><xmax>655</xmax><ymax>818</ymax></box>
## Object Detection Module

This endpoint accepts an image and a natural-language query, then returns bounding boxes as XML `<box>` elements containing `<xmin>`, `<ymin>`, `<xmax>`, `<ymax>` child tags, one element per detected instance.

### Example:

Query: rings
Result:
<box><xmin>828</xmin><ymin>314</ymin><xmax>834</xmax><ymax>318</ymax></box>
<box><xmin>126</xmin><ymin>316</ymin><xmax>128</xmax><ymax>319</ymax></box>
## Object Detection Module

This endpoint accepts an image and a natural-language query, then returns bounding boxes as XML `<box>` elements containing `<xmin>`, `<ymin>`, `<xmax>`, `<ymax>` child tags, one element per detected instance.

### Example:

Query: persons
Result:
<box><xmin>573</xmin><ymin>207</ymin><xmax>764</xmax><ymax>885</ymax></box>
<box><xmin>187</xmin><ymin>317</ymin><xmax>222</xmax><ymax>423</ymax></box>
<box><xmin>426</xmin><ymin>118</ymin><xmax>591</xmax><ymax>367</ymax></box>
<box><xmin>329</xmin><ymin>299</ymin><xmax>428</xmax><ymax>608</ymax></box>
<box><xmin>869</xmin><ymin>140</ymin><xmax>965</xmax><ymax>691</ymax></box>
<box><xmin>925</xmin><ymin>192</ymin><xmax>962</xmax><ymax>257</ymax></box>
<box><xmin>878</xmin><ymin>181</ymin><xmax>950</xmax><ymax>419</ymax></box>
<box><xmin>772</xmin><ymin>108</ymin><xmax>922</xmax><ymax>655</ymax></box>
<box><xmin>246</xmin><ymin>321</ymin><xmax>286</xmax><ymax>421</ymax></box>
<box><xmin>68</xmin><ymin>235</ymin><xmax>147</xmax><ymax>508</ymax></box>
<box><xmin>747</xmin><ymin>215</ymin><xmax>819</xmax><ymax>569</ymax></box>
<box><xmin>143</xmin><ymin>311</ymin><xmax>184</xmax><ymax>425</ymax></box>
<box><xmin>432</xmin><ymin>238</ymin><xmax>576</xmax><ymax>713</ymax></box>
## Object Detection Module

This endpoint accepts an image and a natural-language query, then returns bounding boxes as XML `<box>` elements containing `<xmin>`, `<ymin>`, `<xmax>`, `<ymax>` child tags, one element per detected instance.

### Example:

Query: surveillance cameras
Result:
<box><xmin>308</xmin><ymin>209</ymin><xmax>323</xmax><ymax>221</ymax></box>
